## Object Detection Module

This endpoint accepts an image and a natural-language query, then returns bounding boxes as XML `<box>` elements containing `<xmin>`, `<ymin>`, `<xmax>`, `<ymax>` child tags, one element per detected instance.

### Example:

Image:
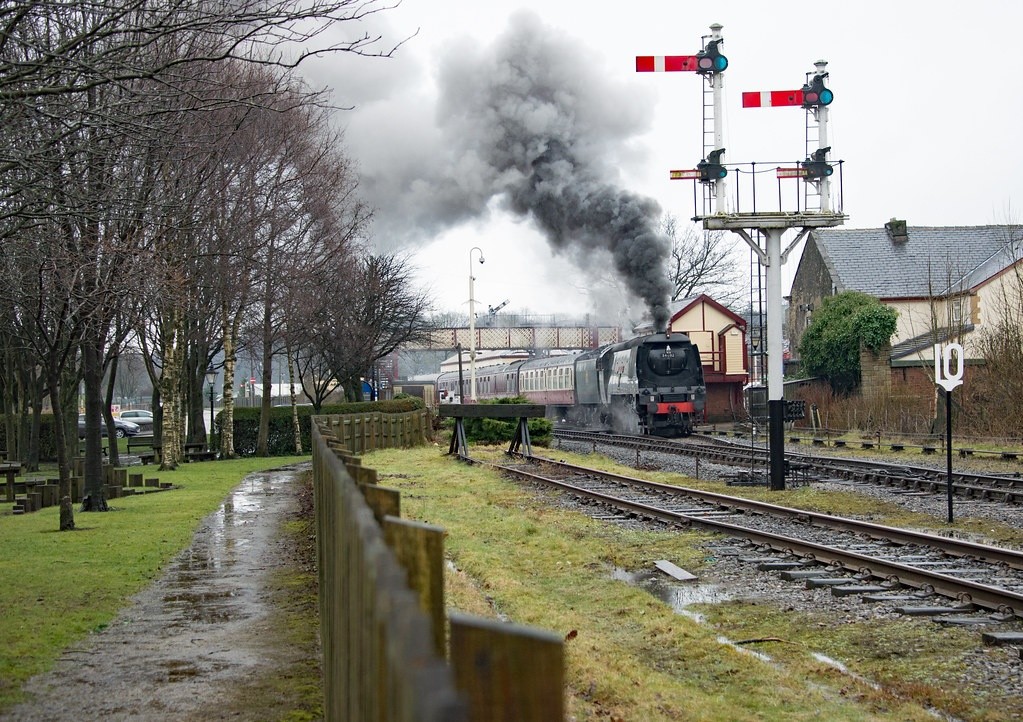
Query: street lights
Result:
<box><xmin>469</xmin><ymin>246</ymin><xmax>486</xmax><ymax>400</ymax></box>
<box><xmin>205</xmin><ymin>362</ymin><xmax>221</xmax><ymax>460</ymax></box>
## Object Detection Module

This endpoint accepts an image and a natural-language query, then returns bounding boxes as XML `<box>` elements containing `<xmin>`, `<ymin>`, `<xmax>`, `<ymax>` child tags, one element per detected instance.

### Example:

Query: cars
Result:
<box><xmin>119</xmin><ymin>410</ymin><xmax>153</xmax><ymax>425</ymax></box>
<box><xmin>77</xmin><ymin>413</ymin><xmax>141</xmax><ymax>438</ymax></box>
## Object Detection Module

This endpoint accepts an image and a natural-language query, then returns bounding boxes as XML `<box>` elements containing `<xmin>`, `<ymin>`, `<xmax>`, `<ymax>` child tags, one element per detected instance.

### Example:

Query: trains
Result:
<box><xmin>435</xmin><ymin>326</ymin><xmax>706</xmax><ymax>436</ymax></box>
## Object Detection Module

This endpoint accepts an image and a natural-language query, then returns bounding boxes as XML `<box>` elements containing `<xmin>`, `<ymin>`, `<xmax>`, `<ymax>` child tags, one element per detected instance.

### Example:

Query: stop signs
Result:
<box><xmin>250</xmin><ymin>377</ymin><xmax>256</xmax><ymax>384</ymax></box>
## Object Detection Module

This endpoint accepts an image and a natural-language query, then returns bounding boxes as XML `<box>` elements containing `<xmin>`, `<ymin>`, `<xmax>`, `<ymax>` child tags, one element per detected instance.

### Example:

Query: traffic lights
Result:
<box><xmin>805</xmin><ymin>147</ymin><xmax>834</xmax><ymax>176</ymax></box>
<box><xmin>805</xmin><ymin>76</ymin><xmax>834</xmax><ymax>107</ymax></box>
<box><xmin>698</xmin><ymin>147</ymin><xmax>727</xmax><ymax>180</ymax></box>
<box><xmin>695</xmin><ymin>40</ymin><xmax>729</xmax><ymax>74</ymax></box>
